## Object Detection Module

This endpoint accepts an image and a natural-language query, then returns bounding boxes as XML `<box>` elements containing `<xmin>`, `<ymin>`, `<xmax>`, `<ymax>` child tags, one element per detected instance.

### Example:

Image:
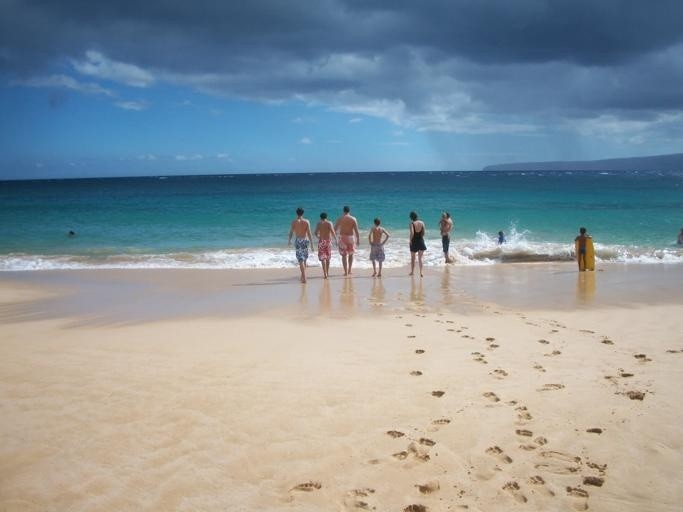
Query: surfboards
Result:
<box><xmin>576</xmin><ymin>234</ymin><xmax>595</xmax><ymax>271</ymax></box>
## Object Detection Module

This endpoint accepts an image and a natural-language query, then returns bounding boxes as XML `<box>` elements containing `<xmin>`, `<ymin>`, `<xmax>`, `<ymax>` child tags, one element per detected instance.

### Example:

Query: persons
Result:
<box><xmin>439</xmin><ymin>213</ymin><xmax>448</xmax><ymax>234</ymax></box>
<box><xmin>408</xmin><ymin>211</ymin><xmax>426</xmax><ymax>277</ymax></box>
<box><xmin>313</xmin><ymin>212</ymin><xmax>338</xmax><ymax>279</ymax></box>
<box><xmin>333</xmin><ymin>206</ymin><xmax>360</xmax><ymax>277</ymax></box>
<box><xmin>288</xmin><ymin>207</ymin><xmax>314</xmax><ymax>283</ymax></box>
<box><xmin>367</xmin><ymin>218</ymin><xmax>390</xmax><ymax>277</ymax></box>
<box><xmin>438</xmin><ymin>211</ymin><xmax>453</xmax><ymax>262</ymax></box>
<box><xmin>497</xmin><ymin>231</ymin><xmax>506</xmax><ymax>245</ymax></box>
<box><xmin>677</xmin><ymin>228</ymin><xmax>683</xmax><ymax>244</ymax></box>
<box><xmin>574</xmin><ymin>227</ymin><xmax>591</xmax><ymax>271</ymax></box>
